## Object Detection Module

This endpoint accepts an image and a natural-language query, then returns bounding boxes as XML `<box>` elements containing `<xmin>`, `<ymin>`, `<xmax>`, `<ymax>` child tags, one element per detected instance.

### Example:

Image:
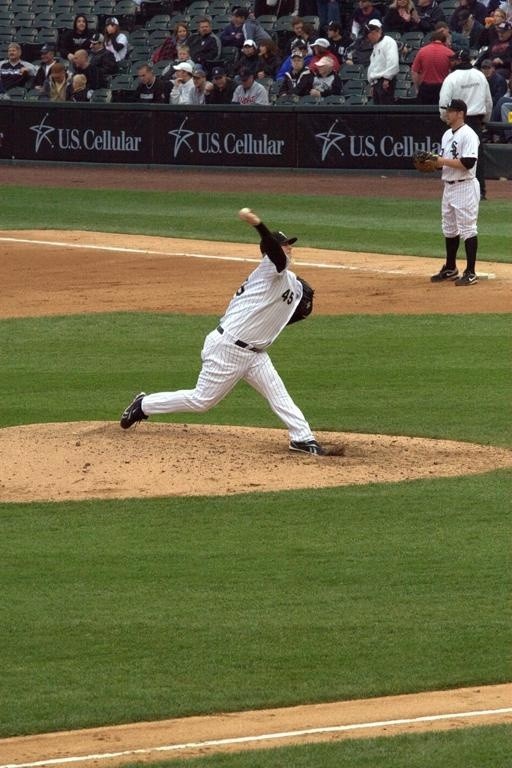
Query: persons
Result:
<box><xmin>2</xmin><ymin>0</ymin><xmax>511</xmax><ymax>143</ymax></box>
<box><xmin>430</xmin><ymin>99</ymin><xmax>481</xmax><ymax>286</ymax></box>
<box><xmin>120</xmin><ymin>208</ymin><xmax>327</xmax><ymax>457</ymax></box>
<box><xmin>437</xmin><ymin>49</ymin><xmax>494</xmax><ymax>200</ymax></box>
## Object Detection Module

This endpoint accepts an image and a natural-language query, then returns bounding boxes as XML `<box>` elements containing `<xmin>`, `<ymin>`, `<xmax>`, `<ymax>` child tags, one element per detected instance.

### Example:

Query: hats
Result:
<box><xmin>291</xmin><ymin>38</ymin><xmax>334</xmax><ymax>67</ymax></box>
<box><xmin>448</xmin><ymin>51</ymin><xmax>468</xmax><ymax>61</ymax></box>
<box><xmin>441</xmin><ymin>100</ymin><xmax>467</xmax><ymax>112</ymax></box>
<box><xmin>174</xmin><ymin>62</ymin><xmax>223</xmax><ymax>79</ymax></box>
<box><xmin>497</xmin><ymin>22</ymin><xmax>510</xmax><ymax>33</ymax></box>
<box><xmin>41</xmin><ymin>46</ymin><xmax>52</xmax><ymax>53</ymax></box>
<box><xmin>482</xmin><ymin>60</ymin><xmax>493</xmax><ymax>68</ymax></box>
<box><xmin>325</xmin><ymin>21</ymin><xmax>339</xmax><ymax>29</ymax></box>
<box><xmin>260</xmin><ymin>231</ymin><xmax>297</xmax><ymax>253</ymax></box>
<box><xmin>91</xmin><ymin>33</ymin><xmax>104</xmax><ymax>44</ymax></box>
<box><xmin>365</xmin><ymin>19</ymin><xmax>382</xmax><ymax>31</ymax></box>
<box><xmin>106</xmin><ymin>17</ymin><xmax>118</xmax><ymax>25</ymax></box>
<box><xmin>244</xmin><ymin>39</ymin><xmax>257</xmax><ymax>48</ymax></box>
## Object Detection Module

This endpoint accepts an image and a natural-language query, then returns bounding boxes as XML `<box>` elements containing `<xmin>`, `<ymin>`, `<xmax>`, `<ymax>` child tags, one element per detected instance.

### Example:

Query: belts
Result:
<box><xmin>218</xmin><ymin>325</ymin><xmax>260</xmax><ymax>352</ymax></box>
<box><xmin>449</xmin><ymin>179</ymin><xmax>464</xmax><ymax>184</ymax></box>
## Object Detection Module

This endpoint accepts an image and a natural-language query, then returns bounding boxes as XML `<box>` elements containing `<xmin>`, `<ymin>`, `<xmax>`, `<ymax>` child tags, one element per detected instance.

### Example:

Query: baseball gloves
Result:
<box><xmin>288</xmin><ymin>276</ymin><xmax>313</xmax><ymax>324</ymax></box>
<box><xmin>414</xmin><ymin>150</ymin><xmax>438</xmax><ymax>172</ymax></box>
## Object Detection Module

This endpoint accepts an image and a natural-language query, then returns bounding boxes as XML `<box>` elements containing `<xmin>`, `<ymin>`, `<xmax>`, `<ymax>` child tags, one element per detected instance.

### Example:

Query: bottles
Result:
<box><xmin>484</xmin><ymin>17</ymin><xmax>496</xmax><ymax>24</ymax></box>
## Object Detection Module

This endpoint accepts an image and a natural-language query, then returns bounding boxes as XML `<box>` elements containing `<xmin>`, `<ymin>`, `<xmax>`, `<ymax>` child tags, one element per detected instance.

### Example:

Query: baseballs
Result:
<box><xmin>240</xmin><ymin>207</ymin><xmax>250</xmax><ymax>213</ymax></box>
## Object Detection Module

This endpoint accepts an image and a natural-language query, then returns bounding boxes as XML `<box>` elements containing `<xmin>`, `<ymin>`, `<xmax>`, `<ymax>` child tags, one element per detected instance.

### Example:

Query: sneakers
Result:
<box><xmin>289</xmin><ymin>441</ymin><xmax>323</xmax><ymax>456</ymax></box>
<box><xmin>456</xmin><ymin>272</ymin><xmax>479</xmax><ymax>286</ymax></box>
<box><xmin>120</xmin><ymin>393</ymin><xmax>148</xmax><ymax>428</ymax></box>
<box><xmin>432</xmin><ymin>265</ymin><xmax>458</xmax><ymax>282</ymax></box>
